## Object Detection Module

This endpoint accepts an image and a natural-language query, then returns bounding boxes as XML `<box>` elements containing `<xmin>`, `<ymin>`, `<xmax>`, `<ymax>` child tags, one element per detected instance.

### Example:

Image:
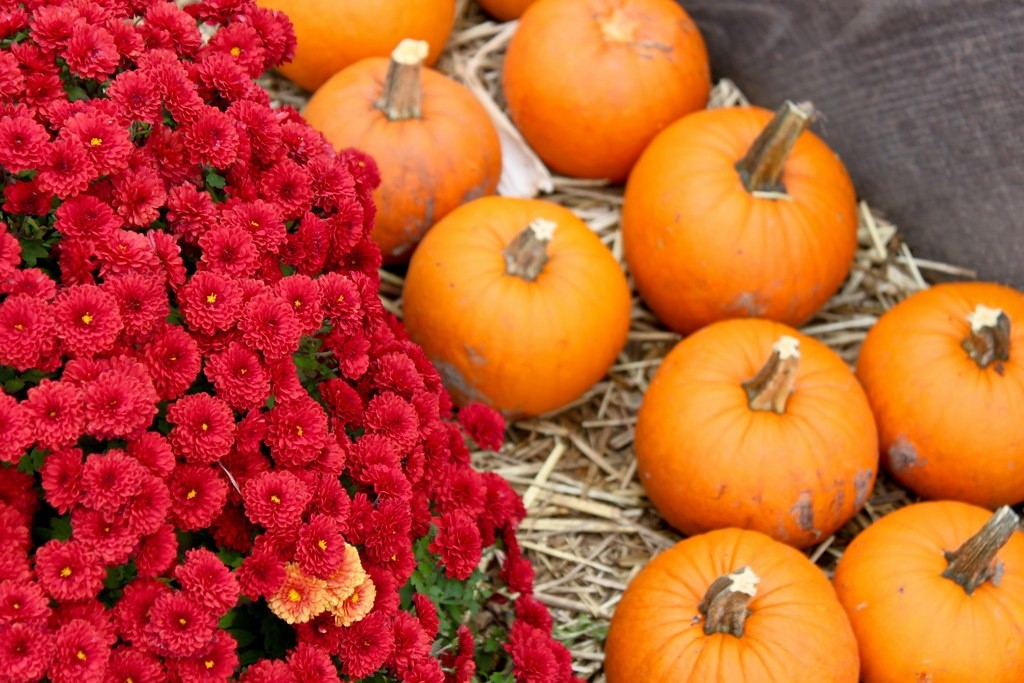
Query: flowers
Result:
<box><xmin>0</xmin><ymin>0</ymin><xmax>588</xmax><ymax>683</ymax></box>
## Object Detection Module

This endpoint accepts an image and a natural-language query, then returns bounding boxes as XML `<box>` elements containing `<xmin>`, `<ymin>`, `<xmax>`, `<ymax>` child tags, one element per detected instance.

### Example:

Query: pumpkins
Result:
<box><xmin>263</xmin><ymin>0</ymin><xmax>1024</xmax><ymax>683</ymax></box>
